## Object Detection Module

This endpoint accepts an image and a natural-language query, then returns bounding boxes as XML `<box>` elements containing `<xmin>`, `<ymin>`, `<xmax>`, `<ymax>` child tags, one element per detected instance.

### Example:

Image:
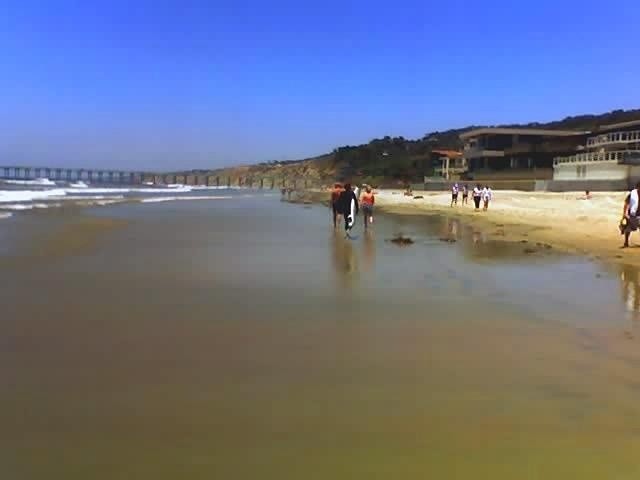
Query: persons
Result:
<box><xmin>462</xmin><ymin>184</ymin><xmax>468</xmax><ymax>207</ymax></box>
<box><xmin>622</xmin><ymin>184</ymin><xmax>640</xmax><ymax>247</ymax></box>
<box><xmin>358</xmin><ymin>185</ymin><xmax>376</xmax><ymax>233</ymax></box>
<box><xmin>339</xmin><ymin>184</ymin><xmax>359</xmax><ymax>240</ymax></box>
<box><xmin>450</xmin><ymin>183</ymin><xmax>458</xmax><ymax>208</ymax></box>
<box><xmin>482</xmin><ymin>184</ymin><xmax>493</xmax><ymax>211</ymax></box>
<box><xmin>471</xmin><ymin>182</ymin><xmax>483</xmax><ymax>211</ymax></box>
<box><xmin>330</xmin><ymin>182</ymin><xmax>344</xmax><ymax>232</ymax></box>
